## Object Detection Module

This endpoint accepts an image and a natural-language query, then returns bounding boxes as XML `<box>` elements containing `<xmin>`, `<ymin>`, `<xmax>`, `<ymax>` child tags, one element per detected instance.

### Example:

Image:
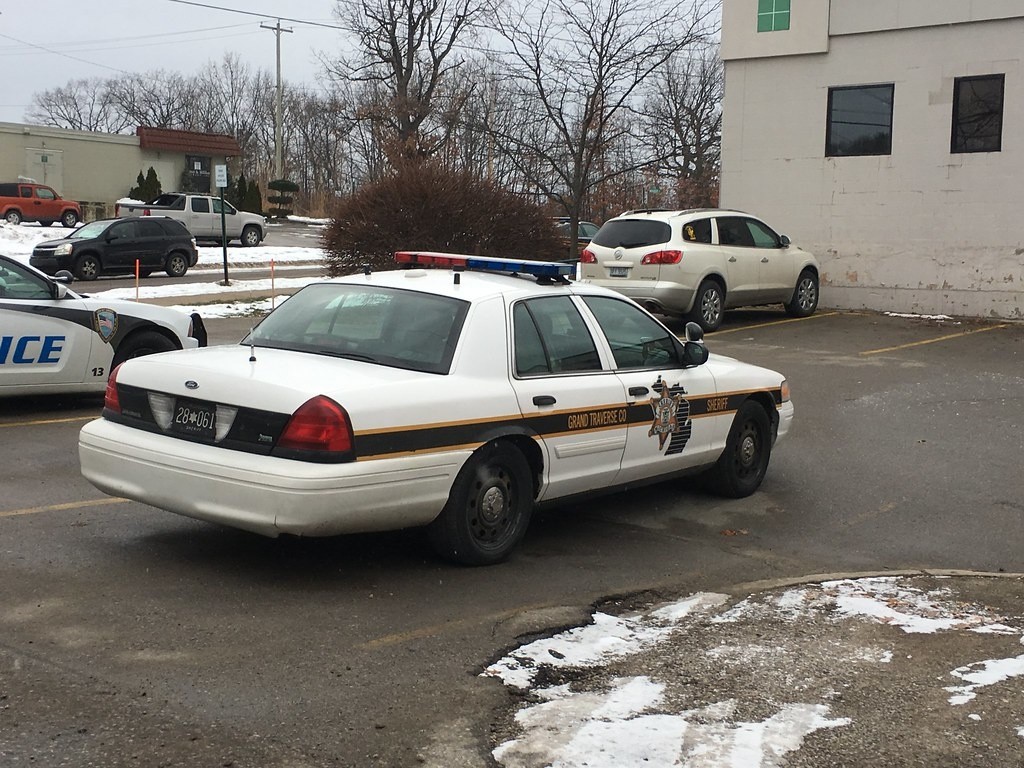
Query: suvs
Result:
<box><xmin>581</xmin><ymin>205</ymin><xmax>823</xmax><ymax>333</ymax></box>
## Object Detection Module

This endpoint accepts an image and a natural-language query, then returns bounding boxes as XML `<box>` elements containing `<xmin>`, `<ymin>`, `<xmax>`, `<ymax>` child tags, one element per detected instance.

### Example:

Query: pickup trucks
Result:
<box><xmin>115</xmin><ymin>191</ymin><xmax>266</xmax><ymax>248</ymax></box>
<box><xmin>0</xmin><ymin>182</ymin><xmax>81</xmax><ymax>227</ymax></box>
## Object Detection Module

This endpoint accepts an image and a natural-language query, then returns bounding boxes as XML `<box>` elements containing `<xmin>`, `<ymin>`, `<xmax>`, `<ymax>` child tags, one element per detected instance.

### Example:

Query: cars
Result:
<box><xmin>0</xmin><ymin>252</ymin><xmax>199</xmax><ymax>399</ymax></box>
<box><xmin>76</xmin><ymin>252</ymin><xmax>795</xmax><ymax>563</ymax></box>
<box><xmin>31</xmin><ymin>215</ymin><xmax>198</xmax><ymax>281</ymax></box>
<box><xmin>551</xmin><ymin>218</ymin><xmax>601</xmax><ymax>249</ymax></box>
